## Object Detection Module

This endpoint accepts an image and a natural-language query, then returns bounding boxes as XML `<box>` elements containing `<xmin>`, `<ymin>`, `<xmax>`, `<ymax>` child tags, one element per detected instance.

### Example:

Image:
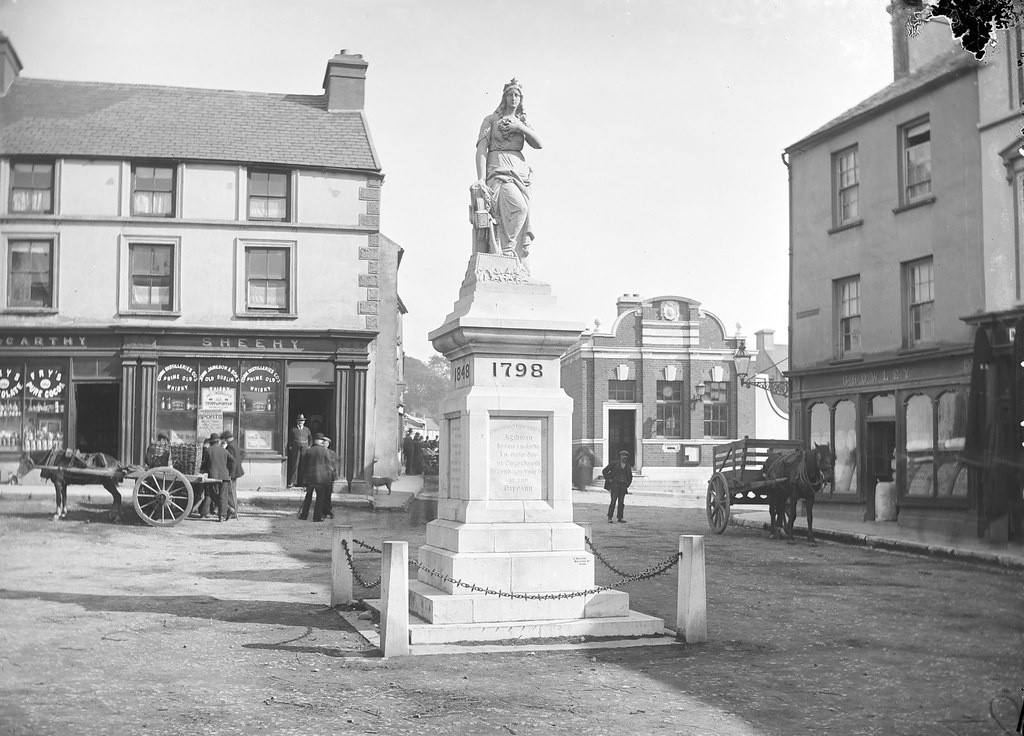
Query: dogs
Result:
<box><xmin>372</xmin><ymin>477</ymin><xmax>394</xmax><ymax>495</ymax></box>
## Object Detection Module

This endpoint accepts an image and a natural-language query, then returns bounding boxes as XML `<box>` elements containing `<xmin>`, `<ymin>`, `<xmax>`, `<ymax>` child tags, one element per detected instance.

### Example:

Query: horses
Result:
<box><xmin>765</xmin><ymin>441</ymin><xmax>836</xmax><ymax>547</ymax></box>
<box><xmin>16</xmin><ymin>449</ymin><xmax>125</xmax><ymax>523</ymax></box>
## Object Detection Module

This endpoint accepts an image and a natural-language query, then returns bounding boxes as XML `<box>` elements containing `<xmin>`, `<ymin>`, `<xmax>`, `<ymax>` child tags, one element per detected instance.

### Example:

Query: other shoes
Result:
<box><xmin>288</xmin><ymin>483</ymin><xmax>293</xmax><ymax>487</ymax></box>
<box><xmin>226</xmin><ymin>506</ymin><xmax>235</xmax><ymax>521</ymax></box>
<box><xmin>608</xmin><ymin>518</ymin><xmax>613</xmax><ymax>523</ymax></box>
<box><xmin>219</xmin><ymin>515</ymin><xmax>226</xmax><ymax>521</ymax></box>
<box><xmin>330</xmin><ymin>510</ymin><xmax>335</xmax><ymax>519</ymax></box>
<box><xmin>313</xmin><ymin>518</ymin><xmax>323</xmax><ymax>522</ymax></box>
<box><xmin>618</xmin><ymin>519</ymin><xmax>626</xmax><ymax>523</ymax></box>
<box><xmin>201</xmin><ymin>513</ymin><xmax>213</xmax><ymax>518</ymax></box>
<box><xmin>298</xmin><ymin>515</ymin><xmax>307</xmax><ymax>520</ymax></box>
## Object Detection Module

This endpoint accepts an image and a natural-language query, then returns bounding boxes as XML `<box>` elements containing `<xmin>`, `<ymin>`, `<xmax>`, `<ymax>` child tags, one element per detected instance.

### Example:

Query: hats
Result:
<box><xmin>295</xmin><ymin>414</ymin><xmax>306</xmax><ymax>421</ymax></box>
<box><xmin>223</xmin><ymin>431</ymin><xmax>233</xmax><ymax>439</ymax></box>
<box><xmin>209</xmin><ymin>433</ymin><xmax>219</xmax><ymax>442</ymax></box>
<box><xmin>323</xmin><ymin>436</ymin><xmax>331</xmax><ymax>443</ymax></box>
<box><xmin>160</xmin><ymin>433</ymin><xmax>169</xmax><ymax>441</ymax></box>
<box><xmin>313</xmin><ymin>433</ymin><xmax>324</xmax><ymax>440</ymax></box>
<box><xmin>204</xmin><ymin>439</ymin><xmax>211</xmax><ymax>443</ymax></box>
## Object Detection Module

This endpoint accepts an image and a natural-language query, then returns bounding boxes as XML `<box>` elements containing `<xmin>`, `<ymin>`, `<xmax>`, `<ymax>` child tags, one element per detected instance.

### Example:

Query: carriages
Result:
<box><xmin>706</xmin><ymin>435</ymin><xmax>837</xmax><ymax>548</ymax></box>
<box><xmin>15</xmin><ymin>442</ymin><xmax>226</xmax><ymax>527</ymax></box>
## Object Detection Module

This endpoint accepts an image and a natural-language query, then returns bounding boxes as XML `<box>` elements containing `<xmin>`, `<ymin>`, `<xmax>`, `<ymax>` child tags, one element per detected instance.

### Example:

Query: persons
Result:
<box><xmin>143</xmin><ymin>429</ymin><xmax>245</xmax><ymax>522</ymax></box>
<box><xmin>471</xmin><ymin>74</ymin><xmax>543</xmax><ymax>261</ymax></box>
<box><xmin>401</xmin><ymin>426</ymin><xmax>440</xmax><ymax>477</ymax></box>
<box><xmin>596</xmin><ymin>450</ymin><xmax>633</xmax><ymax>525</ymax></box>
<box><xmin>286</xmin><ymin>408</ymin><xmax>338</xmax><ymax>523</ymax></box>
